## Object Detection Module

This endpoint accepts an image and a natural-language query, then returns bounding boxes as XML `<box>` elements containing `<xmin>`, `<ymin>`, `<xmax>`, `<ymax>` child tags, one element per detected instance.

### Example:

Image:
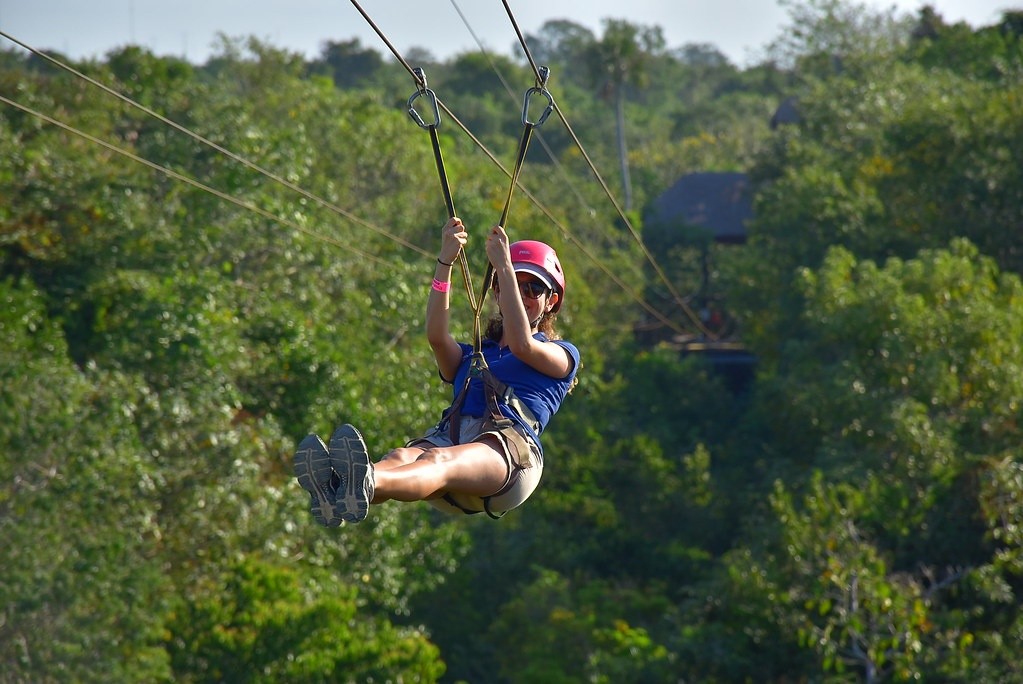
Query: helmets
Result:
<box><xmin>491</xmin><ymin>240</ymin><xmax>565</xmax><ymax>313</ymax></box>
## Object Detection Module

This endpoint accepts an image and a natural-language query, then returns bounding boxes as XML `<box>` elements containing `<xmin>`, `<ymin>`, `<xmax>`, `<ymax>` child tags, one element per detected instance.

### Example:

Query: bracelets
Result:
<box><xmin>437</xmin><ymin>257</ymin><xmax>454</xmax><ymax>267</ymax></box>
<box><xmin>431</xmin><ymin>277</ymin><xmax>452</xmax><ymax>293</ymax></box>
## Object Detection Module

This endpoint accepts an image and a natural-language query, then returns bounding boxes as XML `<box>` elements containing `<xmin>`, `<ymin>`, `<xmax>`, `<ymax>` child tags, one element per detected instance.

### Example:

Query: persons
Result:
<box><xmin>293</xmin><ymin>214</ymin><xmax>581</xmax><ymax>528</ymax></box>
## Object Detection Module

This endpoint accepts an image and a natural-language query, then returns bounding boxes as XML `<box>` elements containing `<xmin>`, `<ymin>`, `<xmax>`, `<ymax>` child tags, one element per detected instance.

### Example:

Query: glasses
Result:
<box><xmin>517</xmin><ymin>280</ymin><xmax>546</xmax><ymax>299</ymax></box>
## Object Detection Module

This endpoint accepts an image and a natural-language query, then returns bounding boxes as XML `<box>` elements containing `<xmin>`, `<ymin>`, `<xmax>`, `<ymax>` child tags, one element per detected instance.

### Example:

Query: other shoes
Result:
<box><xmin>329</xmin><ymin>424</ymin><xmax>376</xmax><ymax>523</ymax></box>
<box><xmin>294</xmin><ymin>434</ymin><xmax>342</xmax><ymax>527</ymax></box>
